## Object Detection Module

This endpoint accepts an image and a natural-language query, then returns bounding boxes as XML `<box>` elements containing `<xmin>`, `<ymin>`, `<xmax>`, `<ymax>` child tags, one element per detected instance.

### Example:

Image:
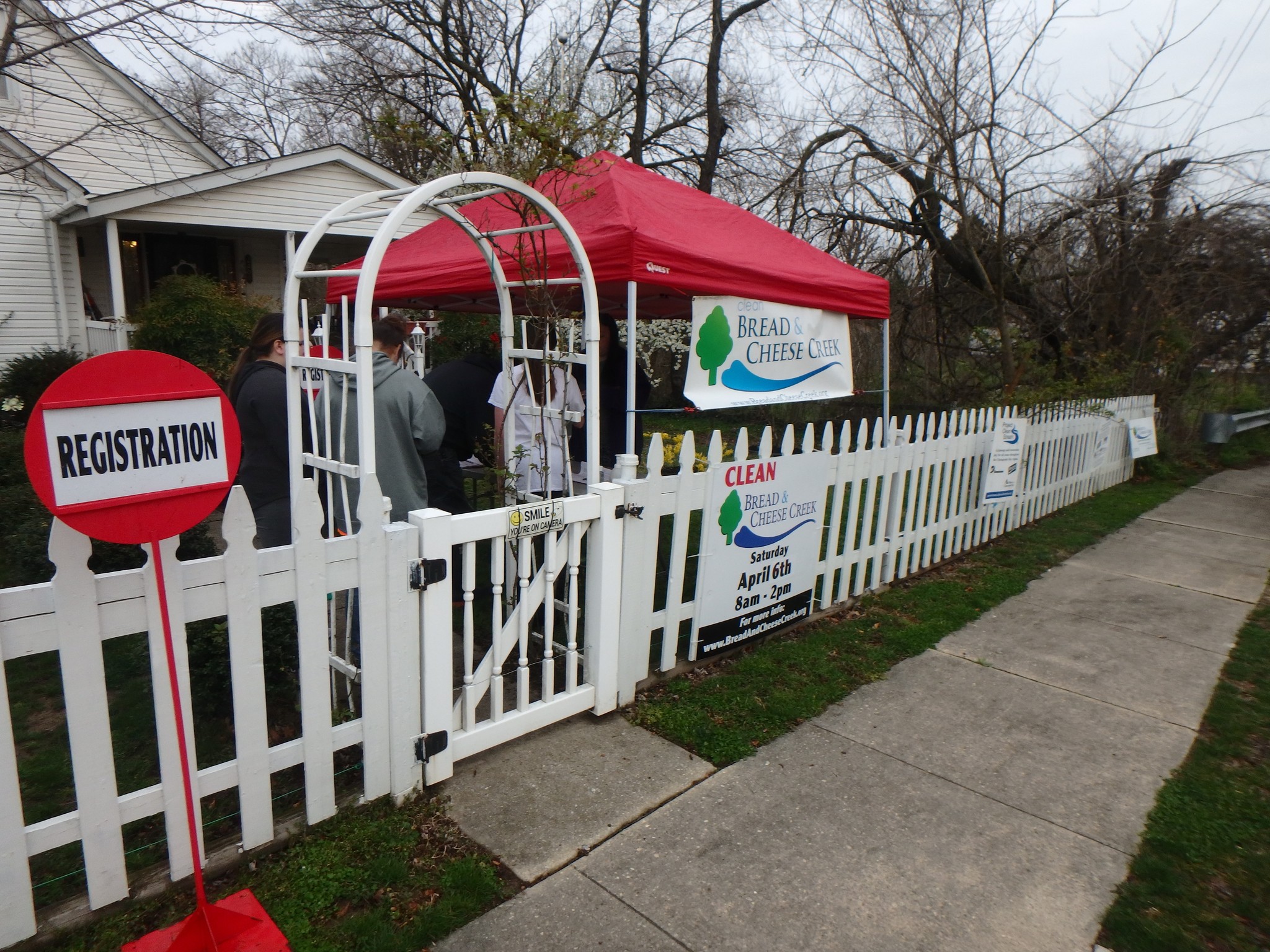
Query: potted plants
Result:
<box><xmin>391</xmin><ymin>307</ymin><xmax>444</xmax><ymax>335</ymax></box>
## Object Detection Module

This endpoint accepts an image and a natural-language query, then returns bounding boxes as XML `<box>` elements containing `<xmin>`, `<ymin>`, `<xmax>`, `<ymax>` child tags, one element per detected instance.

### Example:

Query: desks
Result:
<box><xmin>460</xmin><ymin>460</ymin><xmax>648</xmax><ymax>496</ymax></box>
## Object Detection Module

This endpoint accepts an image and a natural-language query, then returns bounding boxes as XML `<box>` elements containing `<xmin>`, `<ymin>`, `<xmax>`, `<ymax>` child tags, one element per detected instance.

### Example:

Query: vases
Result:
<box><xmin>661</xmin><ymin>466</ymin><xmax>699</xmax><ymax>475</ymax></box>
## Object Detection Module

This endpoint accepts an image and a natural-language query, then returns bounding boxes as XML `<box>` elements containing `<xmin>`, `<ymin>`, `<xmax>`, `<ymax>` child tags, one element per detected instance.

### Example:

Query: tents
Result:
<box><xmin>324</xmin><ymin>148</ymin><xmax>899</xmax><ymax>476</ymax></box>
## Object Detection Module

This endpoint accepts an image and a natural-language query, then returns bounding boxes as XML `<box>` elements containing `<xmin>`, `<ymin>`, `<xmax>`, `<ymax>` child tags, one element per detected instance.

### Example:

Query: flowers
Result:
<box><xmin>642</xmin><ymin>430</ymin><xmax>734</xmax><ymax>473</ymax></box>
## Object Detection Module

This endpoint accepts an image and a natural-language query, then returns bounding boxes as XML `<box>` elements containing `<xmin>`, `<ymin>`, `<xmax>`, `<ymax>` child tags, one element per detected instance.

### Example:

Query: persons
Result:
<box><xmin>415</xmin><ymin>342</ymin><xmax>503</xmax><ymax>605</ymax></box>
<box><xmin>311</xmin><ymin>315</ymin><xmax>448</xmax><ymax>669</ymax></box>
<box><xmin>486</xmin><ymin>316</ymin><xmax>588</xmax><ymax>641</ymax></box>
<box><xmin>225</xmin><ymin>309</ymin><xmax>338</xmax><ymax>626</ymax></box>
<box><xmin>565</xmin><ymin>310</ymin><xmax>649</xmax><ymax>472</ymax></box>
